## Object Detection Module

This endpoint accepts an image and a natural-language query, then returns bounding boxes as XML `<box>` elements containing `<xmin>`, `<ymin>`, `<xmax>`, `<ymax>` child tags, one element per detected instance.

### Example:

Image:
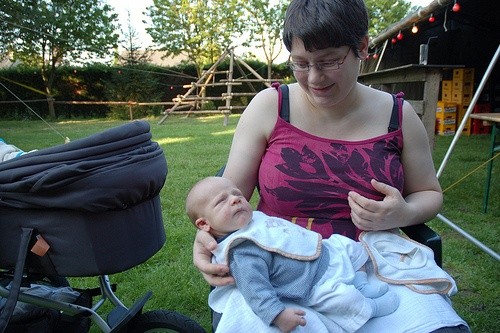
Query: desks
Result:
<box><xmin>356</xmin><ymin>62</ymin><xmax>467</xmax><ymax>154</ymax></box>
<box><xmin>469</xmin><ymin>113</ymin><xmax>500</xmax><ymax>212</ymax></box>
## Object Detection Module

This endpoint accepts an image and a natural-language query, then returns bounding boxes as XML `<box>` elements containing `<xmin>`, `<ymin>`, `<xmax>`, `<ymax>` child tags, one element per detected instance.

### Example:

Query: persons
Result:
<box><xmin>185</xmin><ymin>176</ymin><xmax>400</xmax><ymax>333</ymax></box>
<box><xmin>193</xmin><ymin>0</ymin><xmax>443</xmax><ymax>333</ymax></box>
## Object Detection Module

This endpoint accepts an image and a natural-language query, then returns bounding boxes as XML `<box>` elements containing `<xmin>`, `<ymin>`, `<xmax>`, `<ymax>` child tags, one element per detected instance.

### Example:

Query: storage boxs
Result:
<box><xmin>434</xmin><ymin>68</ymin><xmax>475</xmax><ymax>136</ymax></box>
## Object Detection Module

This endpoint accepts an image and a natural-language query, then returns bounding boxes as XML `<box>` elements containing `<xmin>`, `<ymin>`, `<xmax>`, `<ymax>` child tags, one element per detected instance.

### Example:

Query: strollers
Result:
<box><xmin>0</xmin><ymin>118</ymin><xmax>207</xmax><ymax>333</ymax></box>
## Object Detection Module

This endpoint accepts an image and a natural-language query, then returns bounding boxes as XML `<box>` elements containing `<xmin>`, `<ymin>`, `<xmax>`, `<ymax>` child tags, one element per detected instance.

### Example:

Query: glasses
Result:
<box><xmin>285</xmin><ymin>47</ymin><xmax>351</xmax><ymax>72</ymax></box>
